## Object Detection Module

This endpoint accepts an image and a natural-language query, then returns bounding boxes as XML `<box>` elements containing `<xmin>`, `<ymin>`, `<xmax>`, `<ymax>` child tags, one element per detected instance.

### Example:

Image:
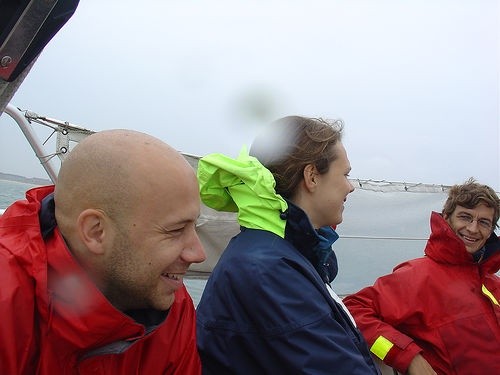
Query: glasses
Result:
<box><xmin>451</xmin><ymin>212</ymin><xmax>500</xmax><ymax>232</ymax></box>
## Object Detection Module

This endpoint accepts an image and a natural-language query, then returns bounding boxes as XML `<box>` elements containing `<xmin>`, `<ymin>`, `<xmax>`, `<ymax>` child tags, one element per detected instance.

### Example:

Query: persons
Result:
<box><xmin>342</xmin><ymin>175</ymin><xmax>500</xmax><ymax>375</ymax></box>
<box><xmin>0</xmin><ymin>129</ymin><xmax>203</xmax><ymax>375</ymax></box>
<box><xmin>197</xmin><ymin>115</ymin><xmax>383</xmax><ymax>375</ymax></box>
